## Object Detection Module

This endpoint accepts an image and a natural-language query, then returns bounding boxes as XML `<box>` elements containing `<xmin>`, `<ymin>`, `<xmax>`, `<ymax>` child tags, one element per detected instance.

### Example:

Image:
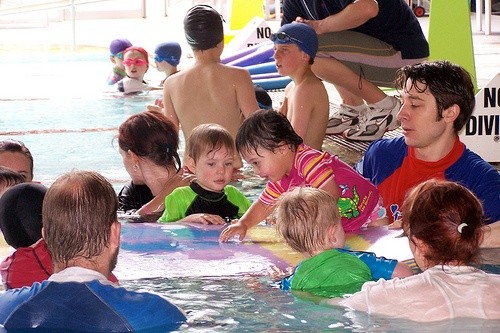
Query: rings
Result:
<box><xmin>189</xmin><ymin>180</ymin><xmax>191</xmax><ymax>183</ymax></box>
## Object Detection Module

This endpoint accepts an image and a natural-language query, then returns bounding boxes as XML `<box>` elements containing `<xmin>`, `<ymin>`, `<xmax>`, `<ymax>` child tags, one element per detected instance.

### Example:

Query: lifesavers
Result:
<box><xmin>218</xmin><ymin>37</ymin><xmax>293</xmax><ymax>87</ymax></box>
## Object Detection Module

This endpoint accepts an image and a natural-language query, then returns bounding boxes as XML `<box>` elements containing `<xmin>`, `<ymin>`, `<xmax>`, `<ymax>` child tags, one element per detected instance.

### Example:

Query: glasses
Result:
<box><xmin>155</xmin><ymin>56</ymin><xmax>177</xmax><ymax>63</ymax></box>
<box><xmin>124</xmin><ymin>58</ymin><xmax>146</xmax><ymax>66</ymax></box>
<box><xmin>116</xmin><ymin>53</ymin><xmax>124</xmax><ymax>59</ymax></box>
<box><xmin>269</xmin><ymin>33</ymin><xmax>305</xmax><ymax>47</ymax></box>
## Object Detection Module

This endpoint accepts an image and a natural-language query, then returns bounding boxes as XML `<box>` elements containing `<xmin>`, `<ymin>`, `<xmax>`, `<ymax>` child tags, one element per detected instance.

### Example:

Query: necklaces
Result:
<box><xmin>153</xmin><ymin>202</ymin><xmax>164</xmax><ymax>213</ymax></box>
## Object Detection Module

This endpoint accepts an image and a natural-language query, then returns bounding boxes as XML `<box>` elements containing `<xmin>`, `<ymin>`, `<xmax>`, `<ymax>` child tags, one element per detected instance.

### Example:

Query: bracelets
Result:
<box><xmin>142</xmin><ymin>205</ymin><xmax>151</xmax><ymax>214</ymax></box>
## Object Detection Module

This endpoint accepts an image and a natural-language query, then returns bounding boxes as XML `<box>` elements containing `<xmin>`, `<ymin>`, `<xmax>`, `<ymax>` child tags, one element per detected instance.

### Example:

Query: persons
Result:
<box><xmin>342</xmin><ymin>179</ymin><xmax>500</xmax><ymax>321</ymax></box>
<box><xmin>356</xmin><ymin>60</ymin><xmax>500</xmax><ymax>250</ymax></box>
<box><xmin>146</xmin><ymin>5</ymin><xmax>258</xmax><ymax>169</ymax></box>
<box><xmin>0</xmin><ymin>138</ymin><xmax>34</xmax><ymax>183</ymax></box>
<box><xmin>154</xmin><ymin>42</ymin><xmax>181</xmax><ymax>85</ymax></box>
<box><xmin>0</xmin><ymin>183</ymin><xmax>118</xmax><ymax>290</ymax></box>
<box><xmin>112</xmin><ymin>112</ymin><xmax>197</xmax><ymax>215</ymax></box>
<box><xmin>270</xmin><ymin>23</ymin><xmax>329</xmax><ymax>151</ymax></box>
<box><xmin>275</xmin><ymin>186</ymin><xmax>412</xmax><ymax>291</ymax></box>
<box><xmin>219</xmin><ymin>107</ymin><xmax>389</xmax><ymax>242</ymax></box>
<box><xmin>157</xmin><ymin>123</ymin><xmax>251</xmax><ymax>225</ymax></box>
<box><xmin>0</xmin><ymin>170</ymin><xmax>188</xmax><ymax>333</ymax></box>
<box><xmin>280</xmin><ymin>0</ymin><xmax>430</xmax><ymax>143</ymax></box>
<box><xmin>0</xmin><ymin>166</ymin><xmax>25</xmax><ymax>199</ymax></box>
<box><xmin>115</xmin><ymin>45</ymin><xmax>163</xmax><ymax>92</ymax></box>
<box><xmin>109</xmin><ymin>39</ymin><xmax>132</xmax><ymax>84</ymax></box>
<box><xmin>240</xmin><ymin>89</ymin><xmax>287</xmax><ymax>126</ymax></box>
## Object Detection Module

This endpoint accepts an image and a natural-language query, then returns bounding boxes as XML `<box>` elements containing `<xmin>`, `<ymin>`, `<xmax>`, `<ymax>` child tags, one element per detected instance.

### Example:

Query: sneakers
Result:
<box><xmin>343</xmin><ymin>94</ymin><xmax>404</xmax><ymax>142</ymax></box>
<box><xmin>325</xmin><ymin>102</ymin><xmax>371</xmax><ymax>134</ymax></box>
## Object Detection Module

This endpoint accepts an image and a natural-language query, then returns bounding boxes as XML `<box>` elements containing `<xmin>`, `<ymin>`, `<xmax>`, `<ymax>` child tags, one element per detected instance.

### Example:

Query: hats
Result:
<box><xmin>274</xmin><ymin>23</ymin><xmax>319</xmax><ymax>58</ymax></box>
<box><xmin>184</xmin><ymin>5</ymin><xmax>223</xmax><ymax>49</ymax></box>
<box><xmin>110</xmin><ymin>40</ymin><xmax>132</xmax><ymax>54</ymax></box>
<box><xmin>154</xmin><ymin>42</ymin><xmax>181</xmax><ymax>66</ymax></box>
